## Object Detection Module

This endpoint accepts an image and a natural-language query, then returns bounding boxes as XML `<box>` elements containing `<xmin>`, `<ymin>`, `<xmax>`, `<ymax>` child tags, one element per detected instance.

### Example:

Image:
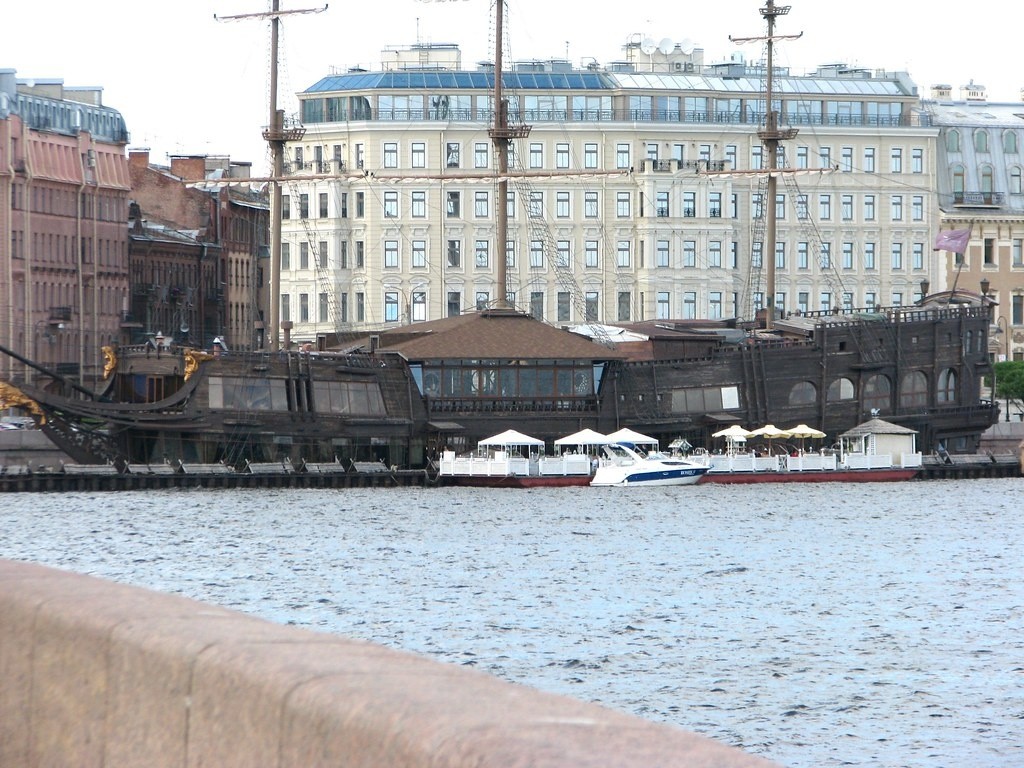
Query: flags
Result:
<box><xmin>933</xmin><ymin>228</ymin><xmax>971</xmax><ymax>255</ymax></box>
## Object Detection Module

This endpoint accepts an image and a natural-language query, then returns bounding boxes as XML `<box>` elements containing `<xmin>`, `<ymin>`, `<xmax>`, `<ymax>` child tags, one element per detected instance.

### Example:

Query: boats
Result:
<box><xmin>589</xmin><ymin>445</ymin><xmax>711</xmax><ymax>487</ymax></box>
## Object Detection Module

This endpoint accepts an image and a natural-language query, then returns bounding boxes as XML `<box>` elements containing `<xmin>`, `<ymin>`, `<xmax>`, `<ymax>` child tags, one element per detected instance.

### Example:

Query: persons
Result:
<box><xmin>790</xmin><ymin>450</ymin><xmax>799</xmax><ymax>457</ymax></box>
<box><xmin>642</xmin><ymin>444</ymin><xmax>648</xmax><ymax>455</ymax></box>
<box><xmin>591</xmin><ymin>456</ymin><xmax>599</xmax><ymax>468</ymax></box>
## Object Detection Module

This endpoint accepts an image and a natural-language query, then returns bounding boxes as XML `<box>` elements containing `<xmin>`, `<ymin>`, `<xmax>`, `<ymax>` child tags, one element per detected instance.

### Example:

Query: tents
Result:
<box><xmin>554</xmin><ymin>428</ymin><xmax>616</xmax><ymax>457</ymax></box>
<box><xmin>477</xmin><ymin>429</ymin><xmax>545</xmax><ymax>459</ymax></box>
<box><xmin>607</xmin><ymin>427</ymin><xmax>660</xmax><ymax>453</ymax></box>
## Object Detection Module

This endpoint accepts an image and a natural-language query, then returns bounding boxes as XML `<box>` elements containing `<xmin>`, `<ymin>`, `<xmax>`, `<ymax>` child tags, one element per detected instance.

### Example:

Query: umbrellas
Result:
<box><xmin>751</xmin><ymin>425</ymin><xmax>789</xmax><ymax>457</ymax></box>
<box><xmin>786</xmin><ymin>424</ymin><xmax>826</xmax><ymax>455</ymax></box>
<box><xmin>712</xmin><ymin>425</ymin><xmax>756</xmax><ymax>439</ymax></box>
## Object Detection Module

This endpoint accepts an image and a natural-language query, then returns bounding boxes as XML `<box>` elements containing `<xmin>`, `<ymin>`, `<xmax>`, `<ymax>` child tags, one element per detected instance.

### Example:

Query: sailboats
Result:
<box><xmin>9</xmin><ymin>0</ymin><xmax>1001</xmax><ymax>464</ymax></box>
